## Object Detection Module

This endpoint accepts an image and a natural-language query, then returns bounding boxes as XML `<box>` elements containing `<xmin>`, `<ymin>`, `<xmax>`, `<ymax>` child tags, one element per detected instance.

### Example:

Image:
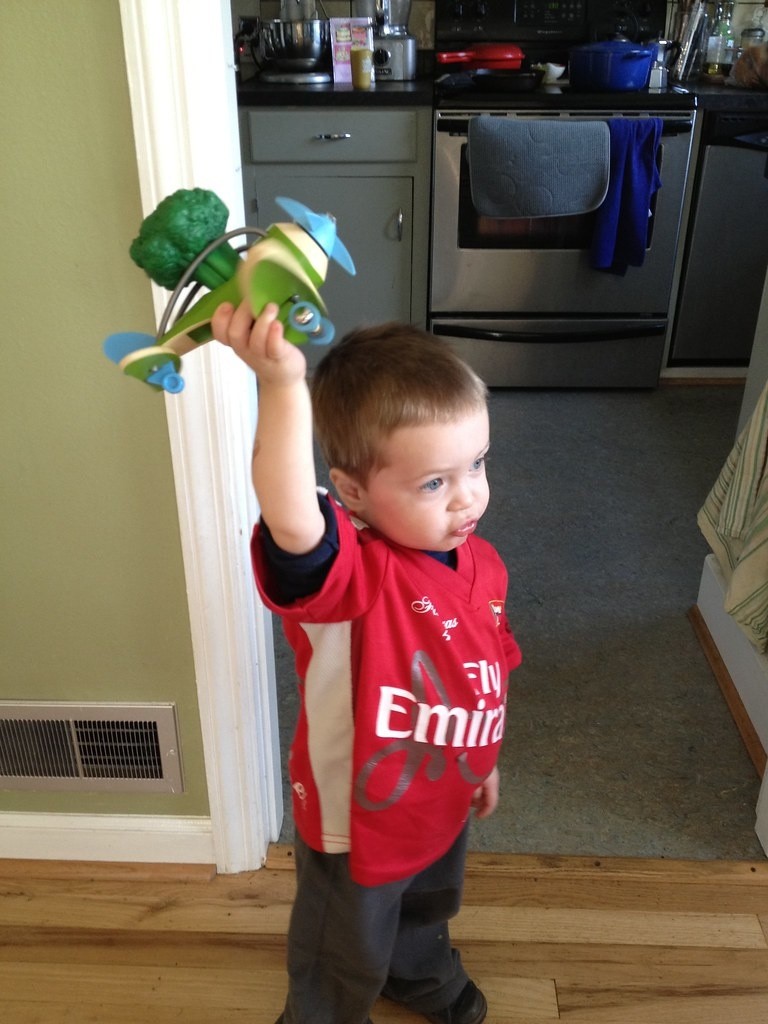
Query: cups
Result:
<box><xmin>350</xmin><ymin>44</ymin><xmax>373</xmax><ymax>89</ymax></box>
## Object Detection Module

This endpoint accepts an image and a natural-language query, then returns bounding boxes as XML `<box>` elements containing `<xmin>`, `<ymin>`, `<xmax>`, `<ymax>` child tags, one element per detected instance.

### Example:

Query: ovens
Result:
<box><xmin>430</xmin><ymin>88</ymin><xmax>697</xmax><ymax>334</ymax></box>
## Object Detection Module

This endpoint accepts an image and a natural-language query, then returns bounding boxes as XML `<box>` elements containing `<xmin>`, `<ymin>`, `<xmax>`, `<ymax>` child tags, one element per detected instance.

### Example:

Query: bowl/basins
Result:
<box><xmin>259</xmin><ymin>17</ymin><xmax>329</xmax><ymax>68</ymax></box>
<box><xmin>464</xmin><ymin>66</ymin><xmax>546</xmax><ymax>93</ymax></box>
<box><xmin>531</xmin><ymin>62</ymin><xmax>565</xmax><ymax>79</ymax></box>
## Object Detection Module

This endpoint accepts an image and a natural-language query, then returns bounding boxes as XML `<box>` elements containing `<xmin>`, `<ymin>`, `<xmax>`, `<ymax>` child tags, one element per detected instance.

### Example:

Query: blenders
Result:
<box><xmin>356</xmin><ymin>0</ymin><xmax>416</xmax><ymax>82</ymax></box>
<box><xmin>256</xmin><ymin>0</ymin><xmax>332</xmax><ymax>83</ymax></box>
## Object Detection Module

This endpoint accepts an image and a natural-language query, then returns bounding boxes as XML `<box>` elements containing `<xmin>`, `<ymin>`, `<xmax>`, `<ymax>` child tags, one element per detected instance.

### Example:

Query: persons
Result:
<box><xmin>213</xmin><ymin>300</ymin><xmax>524</xmax><ymax>1024</ymax></box>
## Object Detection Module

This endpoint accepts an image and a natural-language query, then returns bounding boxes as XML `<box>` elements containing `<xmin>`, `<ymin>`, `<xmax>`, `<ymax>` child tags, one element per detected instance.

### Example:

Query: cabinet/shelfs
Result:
<box><xmin>660</xmin><ymin>108</ymin><xmax>768</xmax><ymax>396</ymax></box>
<box><xmin>241</xmin><ymin>112</ymin><xmax>432</xmax><ymax>380</ymax></box>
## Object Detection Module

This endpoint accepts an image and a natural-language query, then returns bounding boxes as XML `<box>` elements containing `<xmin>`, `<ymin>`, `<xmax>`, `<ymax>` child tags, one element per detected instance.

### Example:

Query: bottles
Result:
<box><xmin>706</xmin><ymin>2</ymin><xmax>735</xmax><ymax>76</ymax></box>
<box><xmin>740</xmin><ymin>27</ymin><xmax>764</xmax><ymax>53</ymax></box>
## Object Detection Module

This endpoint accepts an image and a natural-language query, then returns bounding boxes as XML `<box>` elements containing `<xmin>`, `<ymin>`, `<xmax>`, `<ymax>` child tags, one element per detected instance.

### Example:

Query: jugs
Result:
<box><xmin>647</xmin><ymin>30</ymin><xmax>682</xmax><ymax>87</ymax></box>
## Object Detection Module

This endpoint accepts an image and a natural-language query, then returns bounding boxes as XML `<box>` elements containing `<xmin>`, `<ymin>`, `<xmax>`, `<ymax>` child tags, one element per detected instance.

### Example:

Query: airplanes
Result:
<box><xmin>99</xmin><ymin>187</ymin><xmax>357</xmax><ymax>394</ymax></box>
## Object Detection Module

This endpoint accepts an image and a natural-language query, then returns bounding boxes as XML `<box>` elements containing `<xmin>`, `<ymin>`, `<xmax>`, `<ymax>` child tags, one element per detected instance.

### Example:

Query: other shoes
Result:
<box><xmin>380</xmin><ymin>978</ymin><xmax>487</xmax><ymax>1024</ymax></box>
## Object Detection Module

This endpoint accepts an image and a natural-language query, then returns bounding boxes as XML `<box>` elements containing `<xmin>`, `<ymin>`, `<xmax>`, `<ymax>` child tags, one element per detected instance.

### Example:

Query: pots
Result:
<box><xmin>436</xmin><ymin>42</ymin><xmax>527</xmax><ymax>77</ymax></box>
<box><xmin>559</xmin><ymin>38</ymin><xmax>652</xmax><ymax>92</ymax></box>
